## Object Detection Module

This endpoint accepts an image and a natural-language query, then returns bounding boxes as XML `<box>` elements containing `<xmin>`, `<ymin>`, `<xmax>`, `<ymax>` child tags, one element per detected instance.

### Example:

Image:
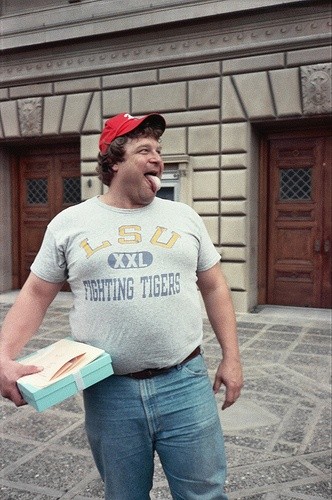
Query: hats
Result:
<box><xmin>99</xmin><ymin>113</ymin><xmax>166</xmax><ymax>153</ymax></box>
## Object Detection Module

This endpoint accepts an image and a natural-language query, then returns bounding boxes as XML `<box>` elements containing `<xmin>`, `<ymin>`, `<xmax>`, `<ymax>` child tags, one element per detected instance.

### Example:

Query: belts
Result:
<box><xmin>122</xmin><ymin>346</ymin><xmax>202</xmax><ymax>379</ymax></box>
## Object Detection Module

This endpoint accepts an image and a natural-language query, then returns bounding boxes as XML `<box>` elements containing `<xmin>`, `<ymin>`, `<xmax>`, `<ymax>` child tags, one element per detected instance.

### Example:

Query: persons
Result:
<box><xmin>0</xmin><ymin>112</ymin><xmax>243</xmax><ymax>500</ymax></box>
